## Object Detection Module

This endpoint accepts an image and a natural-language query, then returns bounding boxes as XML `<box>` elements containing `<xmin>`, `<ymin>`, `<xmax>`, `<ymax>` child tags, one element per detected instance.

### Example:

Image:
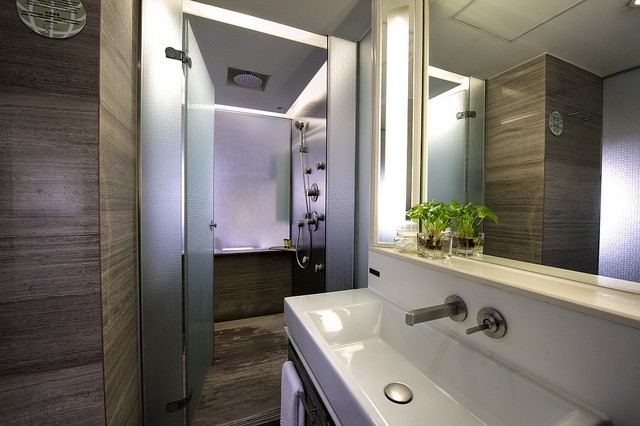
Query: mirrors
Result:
<box><xmin>418</xmin><ymin>0</ymin><xmax>640</xmax><ymax>295</ymax></box>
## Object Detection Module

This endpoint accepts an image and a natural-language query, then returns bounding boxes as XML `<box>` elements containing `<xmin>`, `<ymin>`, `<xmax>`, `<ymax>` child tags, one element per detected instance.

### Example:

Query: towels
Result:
<box><xmin>279</xmin><ymin>362</ymin><xmax>306</xmax><ymax>426</ymax></box>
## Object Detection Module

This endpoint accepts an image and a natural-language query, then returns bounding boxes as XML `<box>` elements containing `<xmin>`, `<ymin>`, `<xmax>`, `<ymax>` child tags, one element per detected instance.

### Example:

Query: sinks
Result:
<box><xmin>282</xmin><ymin>287</ymin><xmax>609</xmax><ymax>425</ymax></box>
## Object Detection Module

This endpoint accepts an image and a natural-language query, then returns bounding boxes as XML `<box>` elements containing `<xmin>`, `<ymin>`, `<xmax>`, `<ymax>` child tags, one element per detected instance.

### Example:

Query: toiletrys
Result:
<box><xmin>287</xmin><ymin>239</ymin><xmax>292</xmax><ymax>249</ymax></box>
<box><xmin>284</xmin><ymin>239</ymin><xmax>288</xmax><ymax>248</ymax></box>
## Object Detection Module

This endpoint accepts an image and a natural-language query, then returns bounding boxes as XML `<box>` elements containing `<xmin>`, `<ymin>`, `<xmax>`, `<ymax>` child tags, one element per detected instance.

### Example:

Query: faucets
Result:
<box><xmin>404</xmin><ymin>295</ymin><xmax>468</xmax><ymax>326</ymax></box>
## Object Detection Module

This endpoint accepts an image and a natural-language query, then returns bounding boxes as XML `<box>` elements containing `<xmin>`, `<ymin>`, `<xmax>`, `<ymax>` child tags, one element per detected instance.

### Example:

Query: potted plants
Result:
<box><xmin>449</xmin><ymin>201</ymin><xmax>498</xmax><ymax>257</ymax></box>
<box><xmin>405</xmin><ymin>200</ymin><xmax>462</xmax><ymax>261</ymax></box>
